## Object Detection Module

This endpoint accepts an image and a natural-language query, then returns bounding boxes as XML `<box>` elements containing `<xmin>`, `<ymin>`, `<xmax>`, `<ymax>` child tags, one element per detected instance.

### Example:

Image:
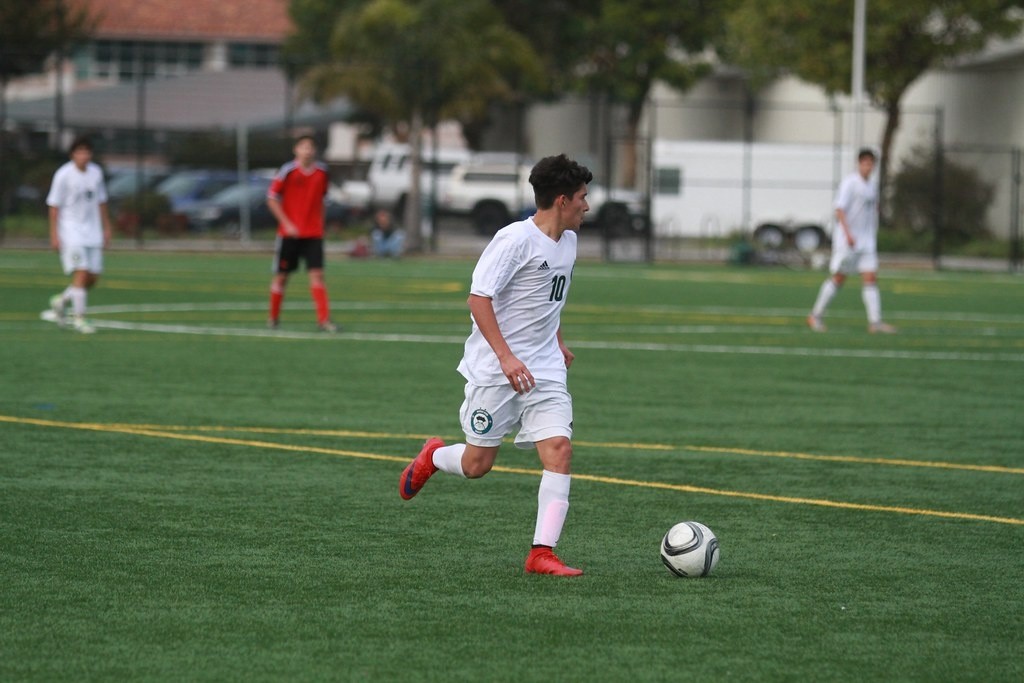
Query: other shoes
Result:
<box><xmin>867</xmin><ymin>321</ymin><xmax>896</xmax><ymax>335</ymax></box>
<box><xmin>73</xmin><ymin>316</ymin><xmax>94</xmax><ymax>333</ymax></box>
<box><xmin>268</xmin><ymin>318</ymin><xmax>277</xmax><ymax>330</ymax></box>
<box><xmin>319</xmin><ymin>320</ymin><xmax>335</xmax><ymax>332</ymax></box>
<box><xmin>48</xmin><ymin>294</ymin><xmax>66</xmax><ymax>328</ymax></box>
<box><xmin>807</xmin><ymin>314</ymin><xmax>827</xmax><ymax>333</ymax></box>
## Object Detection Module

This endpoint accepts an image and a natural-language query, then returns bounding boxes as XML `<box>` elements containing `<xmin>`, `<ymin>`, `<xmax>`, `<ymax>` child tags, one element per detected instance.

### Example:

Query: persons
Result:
<box><xmin>808</xmin><ymin>151</ymin><xmax>897</xmax><ymax>334</ymax></box>
<box><xmin>45</xmin><ymin>140</ymin><xmax>112</xmax><ymax>333</ymax></box>
<box><xmin>267</xmin><ymin>135</ymin><xmax>340</xmax><ymax>333</ymax></box>
<box><xmin>400</xmin><ymin>153</ymin><xmax>595</xmax><ymax>576</ymax></box>
<box><xmin>366</xmin><ymin>120</ymin><xmax>414</xmax><ymax>260</ymax></box>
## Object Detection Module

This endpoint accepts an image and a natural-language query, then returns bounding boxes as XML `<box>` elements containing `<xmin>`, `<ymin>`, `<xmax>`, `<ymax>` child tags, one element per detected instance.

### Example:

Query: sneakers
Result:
<box><xmin>400</xmin><ymin>437</ymin><xmax>447</xmax><ymax>500</ymax></box>
<box><xmin>525</xmin><ymin>546</ymin><xmax>583</xmax><ymax>576</ymax></box>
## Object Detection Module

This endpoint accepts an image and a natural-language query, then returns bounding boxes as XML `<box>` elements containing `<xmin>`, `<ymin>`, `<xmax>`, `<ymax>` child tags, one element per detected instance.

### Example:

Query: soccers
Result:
<box><xmin>660</xmin><ymin>519</ymin><xmax>721</xmax><ymax>579</ymax></box>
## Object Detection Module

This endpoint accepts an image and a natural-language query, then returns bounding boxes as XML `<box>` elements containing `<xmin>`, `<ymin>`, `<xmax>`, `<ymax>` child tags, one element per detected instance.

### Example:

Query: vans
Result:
<box><xmin>647</xmin><ymin>137</ymin><xmax>852</xmax><ymax>249</ymax></box>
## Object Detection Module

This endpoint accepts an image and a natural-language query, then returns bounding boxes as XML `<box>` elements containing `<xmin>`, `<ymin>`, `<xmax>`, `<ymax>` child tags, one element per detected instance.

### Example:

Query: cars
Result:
<box><xmin>368</xmin><ymin>146</ymin><xmax>644</xmax><ymax>230</ymax></box>
<box><xmin>106</xmin><ymin>166</ymin><xmax>352</xmax><ymax>235</ymax></box>
